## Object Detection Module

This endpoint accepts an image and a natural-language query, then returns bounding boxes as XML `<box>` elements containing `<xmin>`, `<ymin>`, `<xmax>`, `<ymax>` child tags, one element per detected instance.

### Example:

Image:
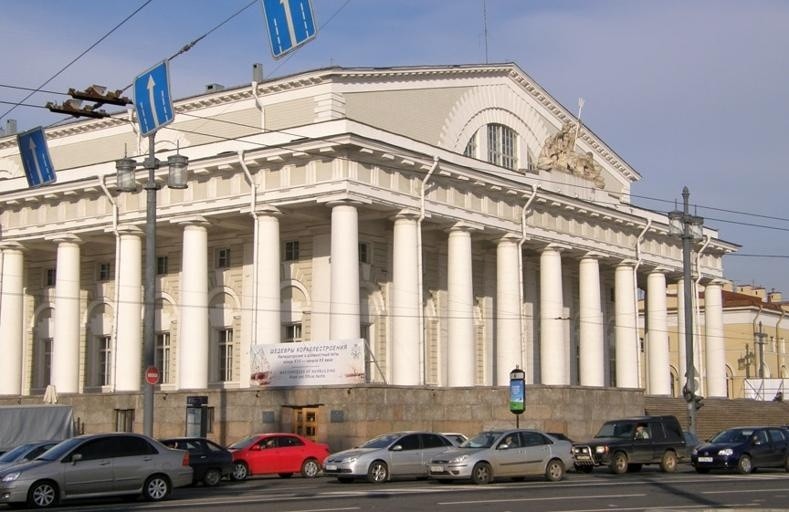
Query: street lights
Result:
<box><xmin>752</xmin><ymin>321</ymin><xmax>769</xmax><ymax>379</ymax></box>
<box><xmin>116</xmin><ymin>138</ymin><xmax>189</xmax><ymax>439</ymax></box>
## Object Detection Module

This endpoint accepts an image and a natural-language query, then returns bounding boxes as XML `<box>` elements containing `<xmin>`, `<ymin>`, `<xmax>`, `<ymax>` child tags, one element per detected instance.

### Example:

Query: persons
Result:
<box><xmin>636</xmin><ymin>426</ymin><xmax>649</xmax><ymax>439</ymax></box>
<box><xmin>505</xmin><ymin>435</ymin><xmax>517</xmax><ymax>449</ymax></box>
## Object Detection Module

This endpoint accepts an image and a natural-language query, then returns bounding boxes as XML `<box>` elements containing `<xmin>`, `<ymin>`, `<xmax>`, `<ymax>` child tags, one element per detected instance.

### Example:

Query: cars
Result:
<box><xmin>437</xmin><ymin>432</ymin><xmax>468</xmax><ymax>448</ymax></box>
<box><xmin>690</xmin><ymin>425</ymin><xmax>789</xmax><ymax>474</ymax></box>
<box><xmin>225</xmin><ymin>434</ymin><xmax>330</xmax><ymax>482</ymax></box>
<box><xmin>322</xmin><ymin>432</ymin><xmax>453</xmax><ymax>485</ymax></box>
<box><xmin>0</xmin><ymin>433</ymin><xmax>192</xmax><ymax>511</ymax></box>
<box><xmin>0</xmin><ymin>439</ymin><xmax>63</xmax><ymax>475</ymax></box>
<box><xmin>546</xmin><ymin>433</ymin><xmax>591</xmax><ymax>474</ymax></box>
<box><xmin>427</xmin><ymin>430</ymin><xmax>574</xmax><ymax>486</ymax></box>
<box><xmin>159</xmin><ymin>437</ymin><xmax>232</xmax><ymax>486</ymax></box>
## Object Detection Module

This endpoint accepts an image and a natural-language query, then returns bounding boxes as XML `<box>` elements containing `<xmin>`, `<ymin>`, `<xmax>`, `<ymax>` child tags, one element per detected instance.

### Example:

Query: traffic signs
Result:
<box><xmin>668</xmin><ymin>186</ymin><xmax>704</xmax><ymax>447</ymax></box>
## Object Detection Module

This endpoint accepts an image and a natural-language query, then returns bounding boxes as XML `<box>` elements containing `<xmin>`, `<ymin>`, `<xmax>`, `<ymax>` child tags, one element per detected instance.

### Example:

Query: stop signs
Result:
<box><xmin>145</xmin><ymin>366</ymin><xmax>159</xmax><ymax>385</ymax></box>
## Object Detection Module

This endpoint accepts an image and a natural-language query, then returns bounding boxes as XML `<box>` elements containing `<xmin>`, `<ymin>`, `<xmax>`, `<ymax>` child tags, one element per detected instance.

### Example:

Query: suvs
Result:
<box><xmin>569</xmin><ymin>415</ymin><xmax>687</xmax><ymax>475</ymax></box>
